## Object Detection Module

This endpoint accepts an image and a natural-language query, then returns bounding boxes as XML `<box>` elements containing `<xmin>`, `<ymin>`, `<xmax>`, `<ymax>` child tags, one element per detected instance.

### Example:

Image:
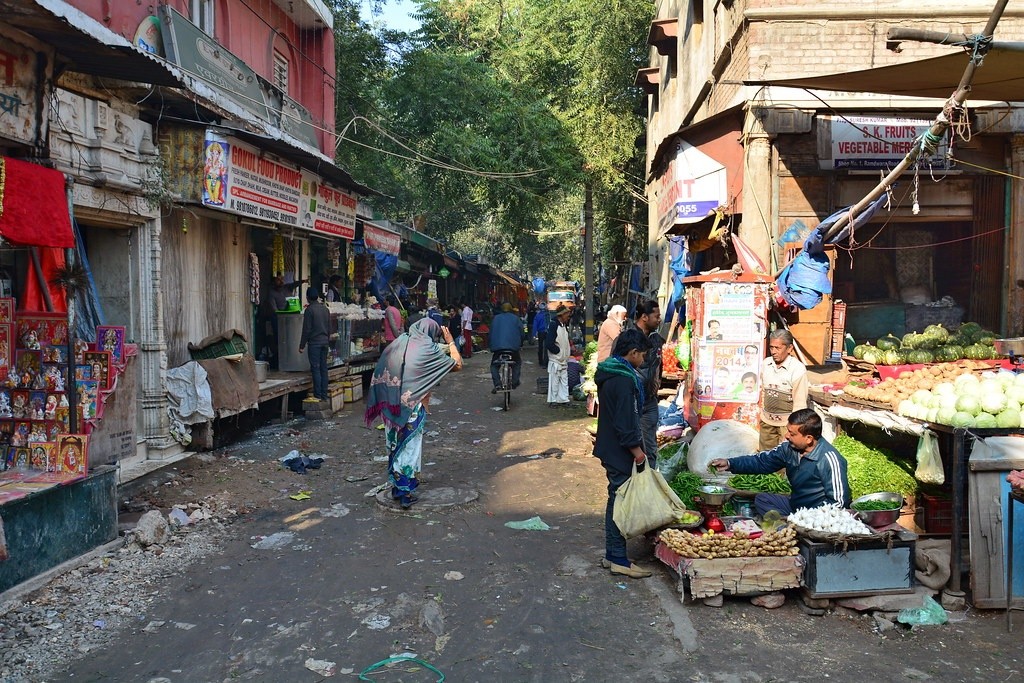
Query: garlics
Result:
<box><xmin>788</xmin><ymin>501</ymin><xmax>872</xmax><ymax>535</ymax></box>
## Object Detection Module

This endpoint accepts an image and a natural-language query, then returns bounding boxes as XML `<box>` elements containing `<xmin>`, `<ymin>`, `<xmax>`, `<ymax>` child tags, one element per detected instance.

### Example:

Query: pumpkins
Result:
<box><xmin>852</xmin><ymin>321</ymin><xmax>1006</xmax><ymax>366</ymax></box>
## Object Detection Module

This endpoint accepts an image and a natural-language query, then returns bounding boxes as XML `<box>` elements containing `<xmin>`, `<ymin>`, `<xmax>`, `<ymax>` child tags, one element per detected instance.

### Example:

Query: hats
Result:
<box><xmin>426</xmin><ymin>298</ymin><xmax>438</xmax><ymax>306</ymax></box>
<box><xmin>538</xmin><ymin>301</ymin><xmax>548</xmax><ymax>310</ymax></box>
<box><xmin>501</xmin><ymin>302</ymin><xmax>512</xmax><ymax>312</ymax></box>
<box><xmin>556</xmin><ymin>305</ymin><xmax>571</xmax><ymax>315</ymax></box>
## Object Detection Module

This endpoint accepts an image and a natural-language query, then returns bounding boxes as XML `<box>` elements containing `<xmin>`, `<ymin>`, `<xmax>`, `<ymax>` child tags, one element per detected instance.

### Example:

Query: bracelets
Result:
<box><xmin>449</xmin><ymin>341</ymin><xmax>455</xmax><ymax>347</ymax></box>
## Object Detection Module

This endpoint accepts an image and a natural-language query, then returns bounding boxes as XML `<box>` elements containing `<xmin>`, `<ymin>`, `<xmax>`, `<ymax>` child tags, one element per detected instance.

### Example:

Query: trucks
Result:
<box><xmin>547</xmin><ymin>282</ymin><xmax>575</xmax><ymax>310</ymax></box>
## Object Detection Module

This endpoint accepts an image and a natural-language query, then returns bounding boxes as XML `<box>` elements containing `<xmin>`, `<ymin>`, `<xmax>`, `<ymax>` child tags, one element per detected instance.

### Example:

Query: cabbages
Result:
<box><xmin>898</xmin><ymin>371</ymin><xmax>1024</xmax><ymax>429</ymax></box>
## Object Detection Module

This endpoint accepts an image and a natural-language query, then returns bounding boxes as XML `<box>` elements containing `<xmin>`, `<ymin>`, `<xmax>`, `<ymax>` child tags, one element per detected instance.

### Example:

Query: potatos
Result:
<box><xmin>842</xmin><ymin>361</ymin><xmax>998</xmax><ymax>411</ymax></box>
<box><xmin>656</xmin><ymin>434</ymin><xmax>677</xmax><ymax>446</ymax></box>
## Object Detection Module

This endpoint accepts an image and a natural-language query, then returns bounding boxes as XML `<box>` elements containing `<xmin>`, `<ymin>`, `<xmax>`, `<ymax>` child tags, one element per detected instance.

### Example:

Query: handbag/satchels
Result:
<box><xmin>913</xmin><ymin>430</ymin><xmax>945</xmax><ymax>485</ymax></box>
<box><xmin>613</xmin><ymin>456</ymin><xmax>686</xmax><ymax>540</ymax></box>
<box><xmin>455</xmin><ymin>335</ymin><xmax>466</xmax><ymax>349</ymax></box>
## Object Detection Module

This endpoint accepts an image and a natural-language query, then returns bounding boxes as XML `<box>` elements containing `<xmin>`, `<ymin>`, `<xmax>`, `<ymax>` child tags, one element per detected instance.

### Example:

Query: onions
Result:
<box><xmin>823</xmin><ymin>380</ymin><xmax>880</xmax><ymax>393</ymax></box>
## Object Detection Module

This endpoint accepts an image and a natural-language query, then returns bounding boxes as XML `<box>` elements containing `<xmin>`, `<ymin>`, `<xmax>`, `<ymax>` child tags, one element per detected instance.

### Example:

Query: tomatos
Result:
<box><xmin>662</xmin><ymin>348</ymin><xmax>681</xmax><ymax>372</ymax></box>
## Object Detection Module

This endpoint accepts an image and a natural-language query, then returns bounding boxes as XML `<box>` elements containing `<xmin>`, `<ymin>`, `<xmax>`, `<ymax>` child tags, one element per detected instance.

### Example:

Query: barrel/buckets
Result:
<box><xmin>255</xmin><ymin>360</ymin><xmax>270</xmax><ymax>383</ymax></box>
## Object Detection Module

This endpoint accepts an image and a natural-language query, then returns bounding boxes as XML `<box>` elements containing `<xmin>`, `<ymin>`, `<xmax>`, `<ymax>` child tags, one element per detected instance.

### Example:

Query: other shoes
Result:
<box><xmin>462</xmin><ymin>355</ymin><xmax>471</xmax><ymax>358</ymax></box>
<box><xmin>512</xmin><ymin>381</ymin><xmax>520</xmax><ymax>389</ymax></box>
<box><xmin>557</xmin><ymin>402</ymin><xmax>572</xmax><ymax>408</ymax></box>
<box><xmin>303</xmin><ymin>397</ymin><xmax>320</xmax><ymax>402</ymax></box>
<box><xmin>401</xmin><ymin>495</ymin><xmax>420</xmax><ymax>507</ymax></box>
<box><xmin>550</xmin><ymin>402</ymin><xmax>559</xmax><ymax>409</ymax></box>
<box><xmin>491</xmin><ymin>382</ymin><xmax>501</xmax><ymax>394</ymax></box>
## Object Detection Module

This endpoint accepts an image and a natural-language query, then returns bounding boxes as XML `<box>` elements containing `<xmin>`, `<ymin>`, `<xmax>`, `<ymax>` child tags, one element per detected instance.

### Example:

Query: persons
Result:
<box><xmin>844</xmin><ymin>251</ymin><xmax>881</xmax><ymax>302</ymax></box>
<box><xmin>759</xmin><ymin>328</ymin><xmax>809</xmax><ymax>479</ymax></box>
<box><xmin>488</xmin><ymin>302</ymin><xmax>526</xmax><ymax>394</ymax></box>
<box><xmin>592</xmin><ymin>299</ymin><xmax>666</xmax><ymax>578</ymax></box>
<box><xmin>695</xmin><ymin>385</ymin><xmax>712</xmax><ymax>398</ymax></box>
<box><xmin>737</xmin><ymin>372</ymin><xmax>758</xmax><ymax>402</ymax></box>
<box><xmin>261</xmin><ymin>271</ymin><xmax>287</xmax><ymax>369</ymax></box>
<box><xmin>0</xmin><ymin>296</ymin><xmax>119</xmax><ymax>476</ymax></box>
<box><xmin>713</xmin><ymin>366</ymin><xmax>730</xmax><ymax>391</ymax></box>
<box><xmin>705</xmin><ymin>319</ymin><xmax>723</xmax><ymax>341</ymax></box>
<box><xmin>706</xmin><ymin>407</ymin><xmax>852</xmax><ymax>522</ymax></box>
<box><xmin>545</xmin><ymin>305</ymin><xmax>572</xmax><ymax>408</ymax></box>
<box><xmin>365</xmin><ymin>317</ymin><xmax>464</xmax><ymax>509</ymax></box>
<box><xmin>744</xmin><ymin>345</ymin><xmax>759</xmax><ymax>367</ymax></box>
<box><xmin>299</xmin><ymin>287</ymin><xmax>332</xmax><ymax>399</ymax></box>
<box><xmin>325</xmin><ymin>273</ymin><xmax>611</xmax><ymax>377</ymax></box>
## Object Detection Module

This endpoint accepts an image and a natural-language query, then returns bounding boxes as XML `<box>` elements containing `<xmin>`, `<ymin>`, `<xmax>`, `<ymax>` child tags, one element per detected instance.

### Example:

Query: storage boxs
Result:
<box><xmin>326</xmin><ymin>383</ymin><xmax>345</xmax><ymax>413</ymax></box>
<box><xmin>537</xmin><ymin>374</ymin><xmax>550</xmax><ymax>395</ymax></box>
<box><xmin>341</xmin><ymin>375</ymin><xmax>364</xmax><ymax>403</ymax></box>
<box><xmin>803</xmin><ymin>525</ymin><xmax>919</xmax><ymax>599</ymax></box>
<box><xmin>920</xmin><ymin>494</ymin><xmax>969</xmax><ymax>533</ymax></box>
<box><xmin>191</xmin><ymin>334</ymin><xmax>248</xmax><ymax>360</ymax></box>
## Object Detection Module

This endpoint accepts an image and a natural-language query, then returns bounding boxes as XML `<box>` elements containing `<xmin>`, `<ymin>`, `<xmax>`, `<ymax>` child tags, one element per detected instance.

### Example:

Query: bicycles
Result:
<box><xmin>493</xmin><ymin>351</ymin><xmax>513</xmax><ymax>411</ymax></box>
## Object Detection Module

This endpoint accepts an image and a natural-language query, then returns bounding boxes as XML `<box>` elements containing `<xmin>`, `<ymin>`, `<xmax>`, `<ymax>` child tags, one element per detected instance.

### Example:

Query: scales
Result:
<box><xmin>694</xmin><ymin>485</ymin><xmax>738</xmax><ymax>532</ymax></box>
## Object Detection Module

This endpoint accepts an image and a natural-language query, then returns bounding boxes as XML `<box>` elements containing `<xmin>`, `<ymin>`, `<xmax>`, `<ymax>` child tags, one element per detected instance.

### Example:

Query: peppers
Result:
<box><xmin>656</xmin><ymin>441</ymin><xmax>689</xmax><ymax>465</ymax></box>
<box><xmin>708</xmin><ymin>462</ymin><xmax>791</xmax><ymax>516</ymax></box>
<box><xmin>668</xmin><ymin>471</ymin><xmax>705</xmax><ymax>510</ymax></box>
<box><xmin>830</xmin><ymin>434</ymin><xmax>916</xmax><ymax>502</ymax></box>
<box><xmin>853</xmin><ymin>499</ymin><xmax>900</xmax><ymax>511</ymax></box>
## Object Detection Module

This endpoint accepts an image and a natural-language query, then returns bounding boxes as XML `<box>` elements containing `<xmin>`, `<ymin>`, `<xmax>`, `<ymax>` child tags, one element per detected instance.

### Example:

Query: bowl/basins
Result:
<box><xmin>849</xmin><ymin>492</ymin><xmax>903</xmax><ymax>527</ymax></box>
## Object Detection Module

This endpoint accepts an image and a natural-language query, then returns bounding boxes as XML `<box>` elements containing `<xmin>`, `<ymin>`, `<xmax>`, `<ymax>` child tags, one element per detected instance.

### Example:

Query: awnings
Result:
<box><xmin>0</xmin><ymin>0</ymin><xmax>396</xmax><ymax>201</ymax></box>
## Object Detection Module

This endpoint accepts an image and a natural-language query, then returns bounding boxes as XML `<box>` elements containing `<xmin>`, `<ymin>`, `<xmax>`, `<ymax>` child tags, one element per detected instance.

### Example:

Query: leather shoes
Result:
<box><xmin>609</xmin><ymin>561</ymin><xmax>653</xmax><ymax>579</ymax></box>
<box><xmin>602</xmin><ymin>557</ymin><xmax>635</xmax><ymax>569</ymax></box>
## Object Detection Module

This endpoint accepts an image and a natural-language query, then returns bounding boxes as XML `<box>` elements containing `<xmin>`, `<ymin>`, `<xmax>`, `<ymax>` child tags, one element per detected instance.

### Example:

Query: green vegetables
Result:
<box><xmin>580</xmin><ymin>339</ymin><xmax>598</xmax><ymax>393</ymax></box>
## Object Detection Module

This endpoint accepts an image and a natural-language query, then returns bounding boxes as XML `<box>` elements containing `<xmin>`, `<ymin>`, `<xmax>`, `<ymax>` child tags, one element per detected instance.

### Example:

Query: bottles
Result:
<box><xmin>742</xmin><ymin>504</ymin><xmax>752</xmax><ymax>518</ymax></box>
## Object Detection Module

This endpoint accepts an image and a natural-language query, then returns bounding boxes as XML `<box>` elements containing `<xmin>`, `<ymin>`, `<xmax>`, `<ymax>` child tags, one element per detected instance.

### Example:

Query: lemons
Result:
<box><xmin>673</xmin><ymin>513</ymin><xmax>699</xmax><ymax>524</ymax></box>
